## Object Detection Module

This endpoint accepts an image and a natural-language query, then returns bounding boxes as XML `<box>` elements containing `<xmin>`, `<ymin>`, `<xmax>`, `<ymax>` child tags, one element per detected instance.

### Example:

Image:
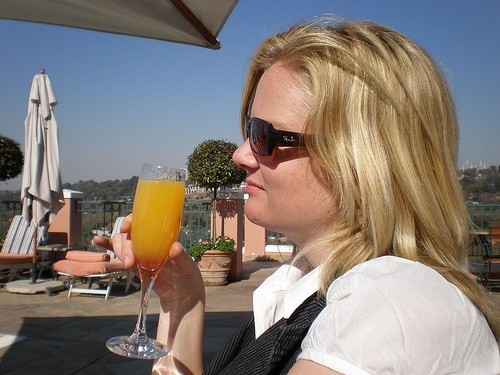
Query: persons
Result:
<box><xmin>93</xmin><ymin>11</ymin><xmax>500</xmax><ymax>375</ymax></box>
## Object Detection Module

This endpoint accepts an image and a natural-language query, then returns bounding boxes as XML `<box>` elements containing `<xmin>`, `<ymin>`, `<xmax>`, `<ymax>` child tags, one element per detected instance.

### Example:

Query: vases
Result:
<box><xmin>195</xmin><ymin>247</ymin><xmax>233</xmax><ymax>288</ymax></box>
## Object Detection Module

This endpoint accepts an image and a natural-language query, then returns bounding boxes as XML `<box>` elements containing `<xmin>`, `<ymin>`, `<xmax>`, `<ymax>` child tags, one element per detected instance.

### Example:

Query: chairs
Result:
<box><xmin>467</xmin><ymin>234</ymin><xmax>500</xmax><ymax>295</ymax></box>
<box><xmin>55</xmin><ymin>216</ymin><xmax>142</xmax><ymax>300</ymax></box>
<box><xmin>0</xmin><ymin>214</ymin><xmax>51</xmax><ymax>286</ymax></box>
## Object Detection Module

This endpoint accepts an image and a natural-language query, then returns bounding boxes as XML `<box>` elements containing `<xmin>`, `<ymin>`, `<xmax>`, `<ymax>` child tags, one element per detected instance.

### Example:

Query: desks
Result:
<box><xmin>35</xmin><ymin>243</ymin><xmax>87</xmax><ymax>281</ymax></box>
<box><xmin>476</xmin><ymin>232</ymin><xmax>500</xmax><ymax>244</ymax></box>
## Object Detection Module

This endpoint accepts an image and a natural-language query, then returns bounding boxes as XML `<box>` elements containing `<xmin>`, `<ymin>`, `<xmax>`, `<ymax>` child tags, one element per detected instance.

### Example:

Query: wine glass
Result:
<box><xmin>106</xmin><ymin>163</ymin><xmax>187</xmax><ymax>360</ymax></box>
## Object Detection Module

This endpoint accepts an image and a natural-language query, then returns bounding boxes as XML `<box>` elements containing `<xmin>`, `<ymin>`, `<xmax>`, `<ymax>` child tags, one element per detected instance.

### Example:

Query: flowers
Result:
<box><xmin>186</xmin><ymin>235</ymin><xmax>236</xmax><ymax>263</ymax></box>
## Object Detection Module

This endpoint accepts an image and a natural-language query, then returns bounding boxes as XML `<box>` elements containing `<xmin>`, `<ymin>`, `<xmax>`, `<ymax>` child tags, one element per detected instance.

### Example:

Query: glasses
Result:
<box><xmin>244</xmin><ymin>115</ymin><xmax>317</xmax><ymax>156</ymax></box>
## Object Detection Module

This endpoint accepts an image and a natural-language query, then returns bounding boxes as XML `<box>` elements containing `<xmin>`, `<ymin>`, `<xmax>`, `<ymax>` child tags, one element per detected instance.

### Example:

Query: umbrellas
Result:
<box><xmin>0</xmin><ymin>0</ymin><xmax>237</xmax><ymax>49</ymax></box>
<box><xmin>21</xmin><ymin>68</ymin><xmax>64</xmax><ymax>284</ymax></box>
